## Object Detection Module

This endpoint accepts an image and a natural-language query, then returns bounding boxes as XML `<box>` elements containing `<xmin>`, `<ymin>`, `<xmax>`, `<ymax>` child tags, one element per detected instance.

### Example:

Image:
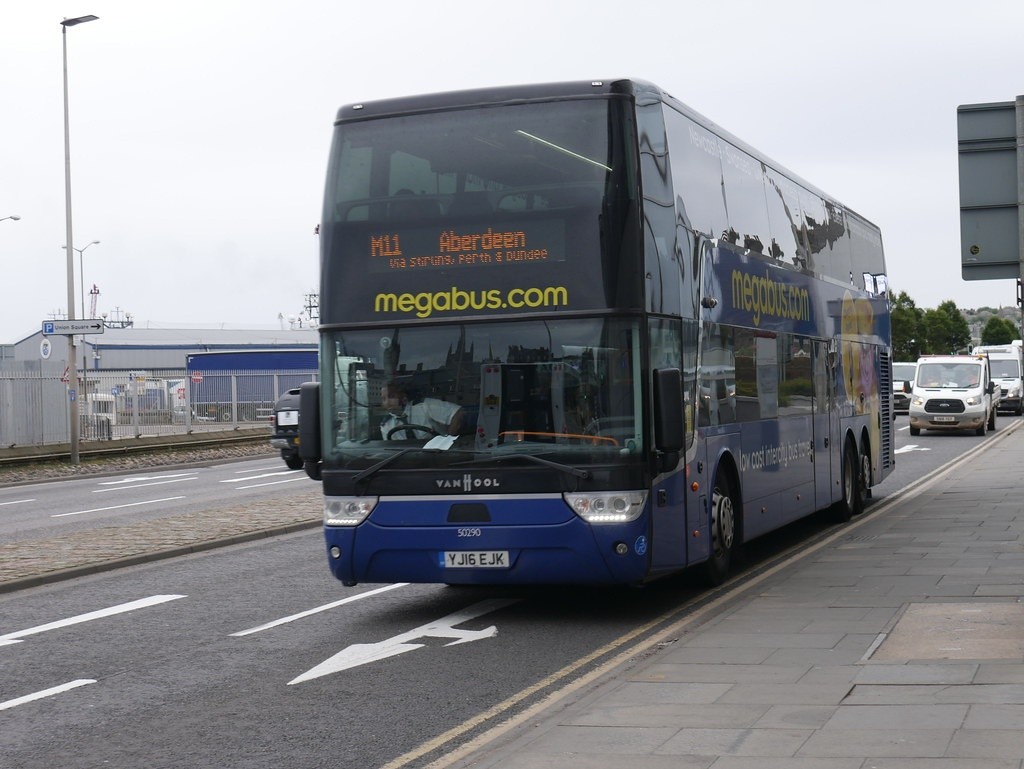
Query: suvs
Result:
<box><xmin>891</xmin><ymin>362</ymin><xmax>917</xmax><ymax>415</ymax></box>
<box><xmin>268</xmin><ymin>382</ymin><xmax>369</xmax><ymax>470</ymax></box>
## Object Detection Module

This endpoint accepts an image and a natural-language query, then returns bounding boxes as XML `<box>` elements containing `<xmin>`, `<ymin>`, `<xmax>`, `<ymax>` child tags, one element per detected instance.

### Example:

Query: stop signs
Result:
<box><xmin>191</xmin><ymin>371</ymin><xmax>203</xmax><ymax>383</ymax></box>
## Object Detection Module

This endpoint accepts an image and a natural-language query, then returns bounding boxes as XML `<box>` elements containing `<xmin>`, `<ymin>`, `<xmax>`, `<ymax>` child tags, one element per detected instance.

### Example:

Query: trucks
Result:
<box><xmin>903</xmin><ymin>350</ymin><xmax>1002</xmax><ymax>437</ymax></box>
<box><xmin>185</xmin><ymin>348</ymin><xmax>369</xmax><ymax>422</ymax></box>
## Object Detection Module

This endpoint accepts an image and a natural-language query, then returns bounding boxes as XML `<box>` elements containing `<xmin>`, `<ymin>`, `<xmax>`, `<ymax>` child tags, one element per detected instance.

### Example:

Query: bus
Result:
<box><xmin>297</xmin><ymin>76</ymin><xmax>894</xmax><ymax>588</ymax></box>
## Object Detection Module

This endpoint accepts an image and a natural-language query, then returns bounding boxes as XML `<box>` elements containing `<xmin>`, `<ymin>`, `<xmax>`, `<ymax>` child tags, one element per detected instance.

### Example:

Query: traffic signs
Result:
<box><xmin>42</xmin><ymin>318</ymin><xmax>105</xmax><ymax>335</ymax></box>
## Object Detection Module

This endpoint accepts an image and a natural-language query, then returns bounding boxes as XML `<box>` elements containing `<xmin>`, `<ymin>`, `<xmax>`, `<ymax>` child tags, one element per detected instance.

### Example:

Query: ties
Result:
<box><xmin>396</xmin><ymin>414</ymin><xmax>417</xmax><ymax>439</ymax></box>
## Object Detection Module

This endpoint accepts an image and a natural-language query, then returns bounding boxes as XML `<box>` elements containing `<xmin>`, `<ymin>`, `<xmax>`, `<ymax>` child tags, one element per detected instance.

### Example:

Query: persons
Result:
<box><xmin>378</xmin><ymin>381</ymin><xmax>464</xmax><ymax>441</ymax></box>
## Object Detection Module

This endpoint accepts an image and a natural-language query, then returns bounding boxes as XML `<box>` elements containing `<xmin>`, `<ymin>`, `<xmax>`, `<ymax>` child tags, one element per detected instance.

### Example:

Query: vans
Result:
<box><xmin>970</xmin><ymin>344</ymin><xmax>1024</xmax><ymax>416</ymax></box>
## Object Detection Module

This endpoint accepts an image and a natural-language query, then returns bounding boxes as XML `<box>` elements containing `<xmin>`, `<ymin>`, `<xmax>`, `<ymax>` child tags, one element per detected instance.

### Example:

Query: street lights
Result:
<box><xmin>59</xmin><ymin>12</ymin><xmax>101</xmax><ymax>466</ymax></box>
<box><xmin>61</xmin><ymin>240</ymin><xmax>100</xmax><ymax>427</ymax></box>
